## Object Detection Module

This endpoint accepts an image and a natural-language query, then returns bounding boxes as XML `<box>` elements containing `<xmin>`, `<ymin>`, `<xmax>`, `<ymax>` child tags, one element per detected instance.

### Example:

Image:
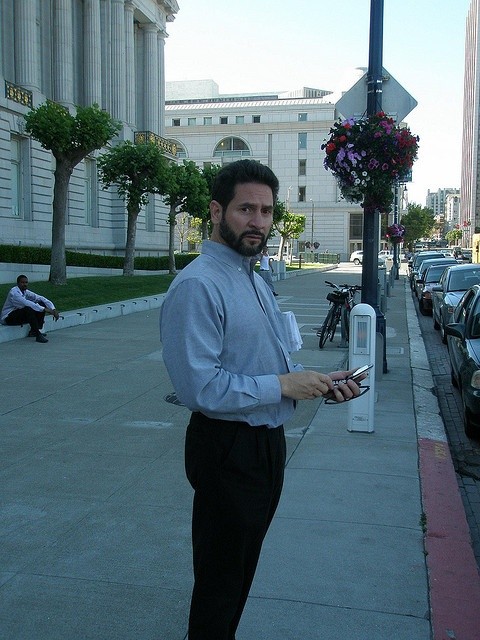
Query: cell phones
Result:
<box><xmin>323</xmin><ymin>364</ymin><xmax>374</xmax><ymax>391</ymax></box>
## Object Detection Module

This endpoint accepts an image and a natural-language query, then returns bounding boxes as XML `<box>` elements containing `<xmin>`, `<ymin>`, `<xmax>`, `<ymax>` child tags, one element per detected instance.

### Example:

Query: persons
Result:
<box><xmin>160</xmin><ymin>160</ymin><xmax>367</xmax><ymax>640</ymax></box>
<box><xmin>0</xmin><ymin>275</ymin><xmax>59</xmax><ymax>343</ymax></box>
<box><xmin>257</xmin><ymin>247</ymin><xmax>279</xmax><ymax>298</ymax></box>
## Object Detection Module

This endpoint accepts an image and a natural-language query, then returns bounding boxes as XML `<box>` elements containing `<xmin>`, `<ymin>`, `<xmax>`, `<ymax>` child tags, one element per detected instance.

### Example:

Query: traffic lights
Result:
<box><xmin>476</xmin><ymin>245</ymin><xmax>479</xmax><ymax>253</ymax></box>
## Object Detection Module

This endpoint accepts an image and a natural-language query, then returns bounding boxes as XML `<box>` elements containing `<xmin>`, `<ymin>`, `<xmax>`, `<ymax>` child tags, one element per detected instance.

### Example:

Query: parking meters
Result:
<box><xmin>347</xmin><ymin>303</ymin><xmax>377</xmax><ymax>434</ymax></box>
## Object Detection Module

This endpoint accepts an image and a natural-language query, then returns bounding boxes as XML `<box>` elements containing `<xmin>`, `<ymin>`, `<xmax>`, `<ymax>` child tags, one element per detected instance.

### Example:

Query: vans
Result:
<box><xmin>415</xmin><ymin>245</ymin><xmax>423</xmax><ymax>252</ymax></box>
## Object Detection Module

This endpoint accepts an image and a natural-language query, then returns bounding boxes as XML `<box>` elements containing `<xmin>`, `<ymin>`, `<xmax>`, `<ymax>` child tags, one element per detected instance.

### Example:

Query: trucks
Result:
<box><xmin>378</xmin><ymin>249</ymin><xmax>406</xmax><ymax>262</ymax></box>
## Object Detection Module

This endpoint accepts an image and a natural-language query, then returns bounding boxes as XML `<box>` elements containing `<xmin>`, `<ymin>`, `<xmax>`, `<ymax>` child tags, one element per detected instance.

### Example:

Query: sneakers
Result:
<box><xmin>36</xmin><ymin>334</ymin><xmax>48</xmax><ymax>343</ymax></box>
<box><xmin>29</xmin><ymin>331</ymin><xmax>47</xmax><ymax>337</ymax></box>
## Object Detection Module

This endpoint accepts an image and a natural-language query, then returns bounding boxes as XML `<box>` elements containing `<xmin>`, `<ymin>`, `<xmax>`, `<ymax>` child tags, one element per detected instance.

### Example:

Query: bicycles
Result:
<box><xmin>316</xmin><ymin>280</ymin><xmax>349</xmax><ymax>348</ymax></box>
<box><xmin>339</xmin><ymin>284</ymin><xmax>362</xmax><ymax>347</ymax></box>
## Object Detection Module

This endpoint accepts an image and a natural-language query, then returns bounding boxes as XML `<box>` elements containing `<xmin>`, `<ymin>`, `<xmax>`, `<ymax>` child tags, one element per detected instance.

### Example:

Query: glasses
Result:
<box><xmin>325</xmin><ymin>383</ymin><xmax>370</xmax><ymax>404</ymax></box>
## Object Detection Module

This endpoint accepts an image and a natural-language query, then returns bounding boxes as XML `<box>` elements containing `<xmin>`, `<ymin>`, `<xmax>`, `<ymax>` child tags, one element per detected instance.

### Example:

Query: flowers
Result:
<box><xmin>320</xmin><ymin>111</ymin><xmax>420</xmax><ymax>214</ymax></box>
<box><xmin>386</xmin><ymin>224</ymin><xmax>406</xmax><ymax>244</ymax></box>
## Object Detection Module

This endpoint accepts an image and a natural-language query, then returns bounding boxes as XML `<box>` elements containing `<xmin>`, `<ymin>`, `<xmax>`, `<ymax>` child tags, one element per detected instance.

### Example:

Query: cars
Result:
<box><xmin>413</xmin><ymin>258</ymin><xmax>459</xmax><ymax>302</ymax></box>
<box><xmin>444</xmin><ymin>285</ymin><xmax>480</xmax><ymax>441</ymax></box>
<box><xmin>350</xmin><ymin>251</ymin><xmax>385</xmax><ymax>266</ymax></box>
<box><xmin>408</xmin><ymin>253</ymin><xmax>445</xmax><ymax>292</ymax></box>
<box><xmin>453</xmin><ymin>246</ymin><xmax>473</xmax><ymax>263</ymax></box>
<box><xmin>416</xmin><ymin>264</ymin><xmax>461</xmax><ymax>315</ymax></box>
<box><xmin>441</xmin><ymin>250</ymin><xmax>451</xmax><ymax>257</ymax></box>
<box><xmin>432</xmin><ymin>264</ymin><xmax>480</xmax><ymax>345</ymax></box>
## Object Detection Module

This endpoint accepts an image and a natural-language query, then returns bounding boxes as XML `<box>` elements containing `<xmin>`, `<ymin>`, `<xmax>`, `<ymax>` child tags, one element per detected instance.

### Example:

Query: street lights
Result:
<box><xmin>309</xmin><ymin>198</ymin><xmax>315</xmax><ymax>263</ymax></box>
<box><xmin>286</xmin><ymin>184</ymin><xmax>294</xmax><ymax>264</ymax></box>
